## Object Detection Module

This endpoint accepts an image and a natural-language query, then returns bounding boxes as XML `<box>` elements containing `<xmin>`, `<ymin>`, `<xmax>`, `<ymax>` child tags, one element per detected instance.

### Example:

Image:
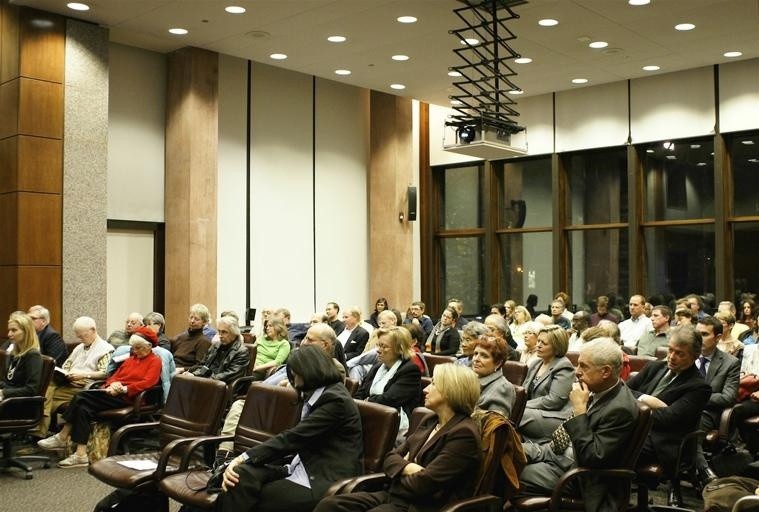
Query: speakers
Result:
<box><xmin>506</xmin><ymin>199</ymin><xmax>528</xmax><ymax>233</ymax></box>
<box><xmin>399</xmin><ymin>184</ymin><xmax>416</xmax><ymax>221</ymax></box>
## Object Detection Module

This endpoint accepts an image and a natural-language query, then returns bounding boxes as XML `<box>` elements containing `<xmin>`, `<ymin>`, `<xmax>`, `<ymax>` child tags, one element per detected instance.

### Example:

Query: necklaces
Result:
<box><xmin>7</xmin><ymin>360</ymin><xmax>21</xmax><ymax>380</ymax></box>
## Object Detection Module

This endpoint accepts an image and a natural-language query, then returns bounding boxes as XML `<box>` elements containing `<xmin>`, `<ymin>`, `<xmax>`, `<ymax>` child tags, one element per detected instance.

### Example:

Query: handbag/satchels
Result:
<box><xmin>735</xmin><ymin>372</ymin><xmax>759</xmax><ymax>402</ymax></box>
<box><xmin>207</xmin><ymin>459</ymin><xmax>233</xmax><ymax>493</ymax></box>
<box><xmin>86</xmin><ymin>420</ymin><xmax>111</xmax><ymax>465</ymax></box>
<box><xmin>122</xmin><ymin>420</ymin><xmax>160</xmax><ymax>454</ymax></box>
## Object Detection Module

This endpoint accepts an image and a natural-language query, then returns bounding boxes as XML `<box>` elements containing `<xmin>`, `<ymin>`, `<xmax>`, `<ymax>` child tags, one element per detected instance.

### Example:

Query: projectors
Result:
<box><xmin>442</xmin><ymin>121</ymin><xmax>527</xmax><ymax>163</ymax></box>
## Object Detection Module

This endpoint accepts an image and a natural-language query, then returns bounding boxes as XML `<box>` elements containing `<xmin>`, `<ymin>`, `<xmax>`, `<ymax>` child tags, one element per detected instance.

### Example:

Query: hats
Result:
<box><xmin>134</xmin><ymin>328</ymin><xmax>159</xmax><ymax>348</ymax></box>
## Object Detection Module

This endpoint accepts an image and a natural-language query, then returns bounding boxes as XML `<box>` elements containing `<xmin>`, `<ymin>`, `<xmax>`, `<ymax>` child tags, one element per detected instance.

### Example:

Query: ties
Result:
<box><xmin>550</xmin><ymin>395</ymin><xmax>593</xmax><ymax>455</ymax></box>
<box><xmin>698</xmin><ymin>357</ymin><xmax>707</xmax><ymax>378</ymax></box>
<box><xmin>657</xmin><ymin>370</ymin><xmax>676</xmax><ymax>388</ymax></box>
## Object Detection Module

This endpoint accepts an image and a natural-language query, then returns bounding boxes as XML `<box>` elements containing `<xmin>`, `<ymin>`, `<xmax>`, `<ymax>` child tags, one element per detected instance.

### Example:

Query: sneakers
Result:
<box><xmin>57</xmin><ymin>452</ymin><xmax>90</xmax><ymax>468</ymax></box>
<box><xmin>37</xmin><ymin>433</ymin><xmax>71</xmax><ymax>451</ymax></box>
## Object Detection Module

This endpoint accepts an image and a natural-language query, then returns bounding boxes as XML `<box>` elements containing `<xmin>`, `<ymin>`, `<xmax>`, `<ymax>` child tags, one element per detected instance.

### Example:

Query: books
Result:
<box><xmin>53</xmin><ymin>366</ymin><xmax>69</xmax><ymax>386</ymax></box>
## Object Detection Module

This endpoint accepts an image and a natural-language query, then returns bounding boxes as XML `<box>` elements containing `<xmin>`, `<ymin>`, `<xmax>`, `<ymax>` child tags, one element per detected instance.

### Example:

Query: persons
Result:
<box><xmin>36</xmin><ymin>328</ymin><xmax>162</xmax><ymax>469</ymax></box>
<box><xmin>28</xmin><ymin>316</ymin><xmax>117</xmax><ymax>438</ymax></box>
<box><xmin>107</xmin><ymin>291</ymin><xmax>758</xmax><ymax>512</ymax></box>
<box><xmin>27</xmin><ymin>305</ymin><xmax>68</xmax><ymax>368</ymax></box>
<box><xmin>0</xmin><ymin>311</ymin><xmax>42</xmax><ymax>401</ymax></box>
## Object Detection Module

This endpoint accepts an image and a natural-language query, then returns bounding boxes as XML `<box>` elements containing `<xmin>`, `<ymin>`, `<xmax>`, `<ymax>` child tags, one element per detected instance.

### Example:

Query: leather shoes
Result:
<box><xmin>699</xmin><ymin>466</ymin><xmax>718</xmax><ymax>486</ymax></box>
<box><xmin>666</xmin><ymin>485</ymin><xmax>683</xmax><ymax>506</ymax></box>
<box><xmin>15</xmin><ymin>447</ymin><xmax>42</xmax><ymax>455</ymax></box>
